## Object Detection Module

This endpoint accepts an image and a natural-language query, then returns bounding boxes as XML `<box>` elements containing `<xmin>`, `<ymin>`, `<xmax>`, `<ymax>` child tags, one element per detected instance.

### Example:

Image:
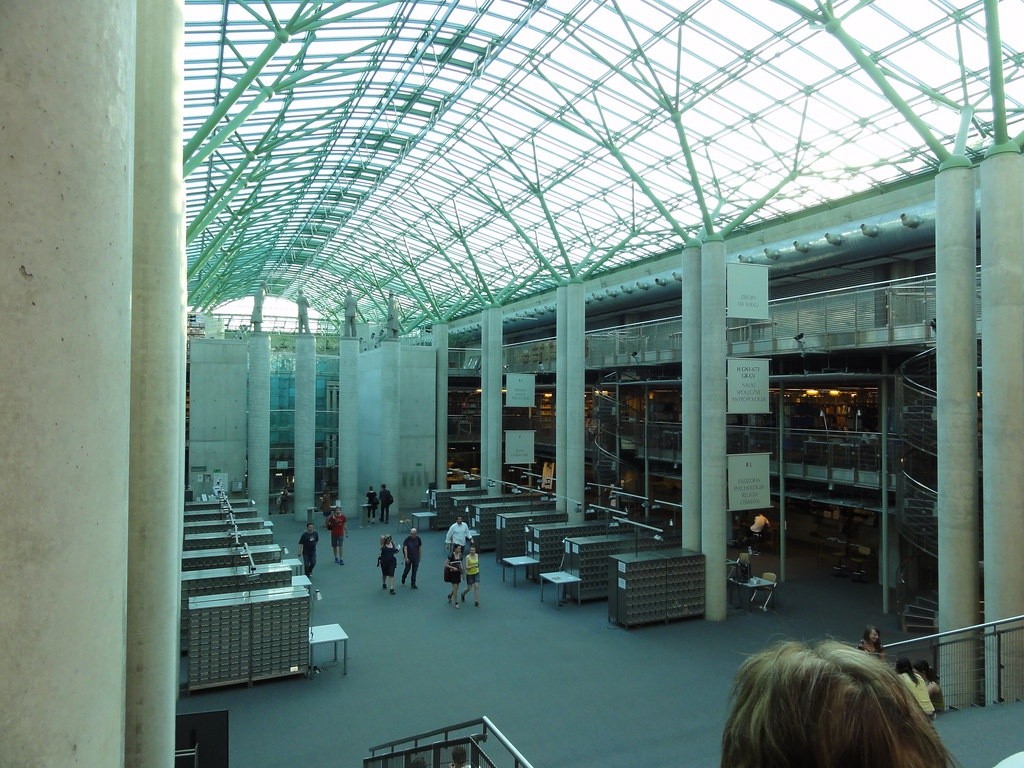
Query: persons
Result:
<box><xmin>250</xmin><ymin>280</ymin><xmax>266</xmax><ymax>333</ymax></box>
<box><xmin>378</xmin><ymin>483</ymin><xmax>393</xmax><ymax>524</ymax></box>
<box><xmin>298</xmin><ymin>522</ymin><xmax>319</xmax><ymax>579</ymax></box>
<box><xmin>719</xmin><ymin>638</ymin><xmax>965</xmax><ymax>768</ymax></box>
<box><xmin>443</xmin><ymin>544</ymin><xmax>465</xmax><ymax>609</ymax></box>
<box><xmin>742</xmin><ymin>510</ymin><xmax>770</xmax><ymax>542</ymax></box>
<box><xmin>401</xmin><ymin>527</ymin><xmax>423</xmax><ymax>589</ymax></box>
<box><xmin>343</xmin><ymin>290</ymin><xmax>358</xmax><ymax>337</ymax></box>
<box><xmin>366</xmin><ymin>485</ymin><xmax>380</xmax><ymax>524</ymax></box>
<box><xmin>296</xmin><ymin>288</ymin><xmax>311</xmax><ymax>334</ymax></box>
<box><xmin>894</xmin><ymin>657</ymin><xmax>937</xmax><ymax>721</ymax></box>
<box><xmin>461</xmin><ymin>545</ymin><xmax>481</xmax><ymax>608</ymax></box>
<box><xmin>913</xmin><ymin>659</ymin><xmax>945</xmax><ymax>714</ymax></box>
<box><xmin>855</xmin><ymin>623</ymin><xmax>888</xmax><ymax>663</ymax></box>
<box><xmin>318</xmin><ymin>493</ymin><xmax>331</xmax><ymax>528</ymax></box>
<box><xmin>444</xmin><ymin>516</ymin><xmax>475</xmax><ymax>557</ymax></box>
<box><xmin>327</xmin><ymin>505</ymin><xmax>348</xmax><ymax>566</ymax></box>
<box><xmin>406</xmin><ymin>755</ymin><xmax>427</xmax><ymax>768</ymax></box>
<box><xmin>385</xmin><ymin>291</ymin><xmax>400</xmax><ymax>340</ymax></box>
<box><xmin>448</xmin><ymin>747</ymin><xmax>472</xmax><ymax>768</ymax></box>
<box><xmin>279</xmin><ymin>483</ymin><xmax>293</xmax><ymax>514</ymax></box>
<box><xmin>376</xmin><ymin>534</ymin><xmax>401</xmax><ymax>594</ymax></box>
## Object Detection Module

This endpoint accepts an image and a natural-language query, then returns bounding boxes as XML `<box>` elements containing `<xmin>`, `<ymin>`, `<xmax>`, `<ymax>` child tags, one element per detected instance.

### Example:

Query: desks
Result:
<box><xmin>281</xmin><ymin>557</ymin><xmax>349</xmax><ymax>679</ymax></box>
<box><xmin>502</xmin><ymin>556</ymin><xmax>540</xmax><ymax>588</ymax></box>
<box><xmin>412</xmin><ymin>512</ymin><xmax>437</xmax><ymax>533</ymax></box>
<box><xmin>264</xmin><ymin>520</ymin><xmax>274</xmax><ymax>530</ymax></box>
<box><xmin>540</xmin><ymin>571</ymin><xmax>582</xmax><ymax>610</ymax></box>
<box><xmin>467</xmin><ymin>530</ymin><xmax>480</xmax><ymax>554</ymax></box>
<box><xmin>728</xmin><ymin>576</ymin><xmax>776</xmax><ymax>612</ymax></box>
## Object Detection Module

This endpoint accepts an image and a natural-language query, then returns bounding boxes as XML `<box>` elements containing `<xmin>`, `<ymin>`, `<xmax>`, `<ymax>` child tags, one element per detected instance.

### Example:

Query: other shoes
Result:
<box><xmin>390</xmin><ymin>589</ymin><xmax>395</xmax><ymax>594</ymax></box>
<box><xmin>411</xmin><ymin>584</ymin><xmax>417</xmax><ymax>589</ymax></box>
<box><xmin>335</xmin><ymin>557</ymin><xmax>339</xmax><ymax>563</ymax></box>
<box><xmin>475</xmin><ymin>601</ymin><xmax>481</xmax><ymax>607</ymax></box>
<box><xmin>383</xmin><ymin>584</ymin><xmax>387</xmax><ymax>588</ymax></box>
<box><xmin>448</xmin><ymin>594</ymin><xmax>452</xmax><ymax>603</ymax></box>
<box><xmin>461</xmin><ymin>592</ymin><xmax>465</xmax><ymax>601</ymax></box>
<box><xmin>340</xmin><ymin>559</ymin><xmax>344</xmax><ymax>565</ymax></box>
<box><xmin>454</xmin><ymin>602</ymin><xmax>461</xmax><ymax>609</ymax></box>
<box><xmin>402</xmin><ymin>576</ymin><xmax>405</xmax><ymax>584</ymax></box>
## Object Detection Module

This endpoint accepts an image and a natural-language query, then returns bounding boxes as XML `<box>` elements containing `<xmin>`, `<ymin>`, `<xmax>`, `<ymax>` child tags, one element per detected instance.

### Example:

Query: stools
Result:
<box><xmin>749</xmin><ymin>529</ymin><xmax>762</xmax><ymax>556</ymax></box>
<box><xmin>833</xmin><ymin>552</ymin><xmax>848</xmax><ymax>577</ymax></box>
<box><xmin>852</xmin><ymin>557</ymin><xmax>866</xmax><ymax>583</ymax></box>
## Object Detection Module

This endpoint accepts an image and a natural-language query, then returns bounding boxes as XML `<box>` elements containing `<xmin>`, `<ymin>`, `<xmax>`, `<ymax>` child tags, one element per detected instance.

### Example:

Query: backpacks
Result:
<box><xmin>384</xmin><ymin>491</ymin><xmax>393</xmax><ymax>504</ymax></box>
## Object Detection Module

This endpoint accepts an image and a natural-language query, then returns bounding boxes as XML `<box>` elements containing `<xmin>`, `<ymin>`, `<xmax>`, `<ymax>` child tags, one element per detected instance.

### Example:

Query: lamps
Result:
<box><xmin>465</xmin><ymin>505</ymin><xmax>469</xmax><ymax>513</ymax></box>
<box><xmin>316</xmin><ymin>590</ymin><xmax>322</xmax><ymax>600</ymax></box>
<box><xmin>463</xmin><ymin>473</ymin><xmax>664</xmax><ymax>541</ymax></box>
<box><xmin>283</xmin><ymin>546</ymin><xmax>288</xmax><ymax>554</ymax></box>
<box><xmin>220</xmin><ymin>492</ymin><xmax>259</xmax><ymax>579</ymax></box>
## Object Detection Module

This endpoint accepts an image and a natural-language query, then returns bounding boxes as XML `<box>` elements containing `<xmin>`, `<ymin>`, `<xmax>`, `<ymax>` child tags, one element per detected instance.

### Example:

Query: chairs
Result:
<box><xmin>399</xmin><ymin>512</ymin><xmax>412</xmax><ymax>532</ymax></box>
<box><xmin>751</xmin><ymin>572</ymin><xmax>777</xmax><ymax>608</ymax></box>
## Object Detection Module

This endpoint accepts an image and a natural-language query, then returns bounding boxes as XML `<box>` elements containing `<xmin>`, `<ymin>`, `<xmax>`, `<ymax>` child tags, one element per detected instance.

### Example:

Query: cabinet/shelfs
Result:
<box><xmin>179</xmin><ymin>499</ymin><xmax>311</xmax><ymax>696</ymax></box>
<box><xmin>431</xmin><ymin>490</ymin><xmax>706</xmax><ymax>630</ymax></box>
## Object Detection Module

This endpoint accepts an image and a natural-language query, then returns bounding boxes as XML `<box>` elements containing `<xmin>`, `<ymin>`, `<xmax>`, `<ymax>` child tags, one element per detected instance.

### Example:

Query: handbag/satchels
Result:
<box><xmin>371</xmin><ymin>496</ymin><xmax>379</xmax><ymax>505</ymax></box>
<box><xmin>444</xmin><ymin>568</ymin><xmax>451</xmax><ymax>582</ymax></box>
